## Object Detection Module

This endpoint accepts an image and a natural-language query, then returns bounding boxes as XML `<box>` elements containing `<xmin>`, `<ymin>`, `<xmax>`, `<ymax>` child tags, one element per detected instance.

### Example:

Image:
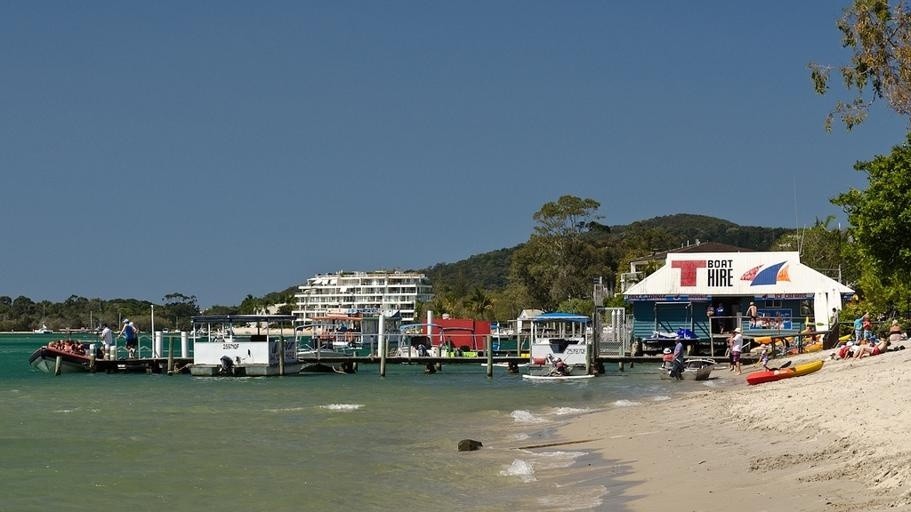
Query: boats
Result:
<box><xmin>295</xmin><ymin>324</ymin><xmax>346</xmax><ymax>374</ymax></box>
<box><xmin>395</xmin><ymin>324</ymin><xmax>478</xmax><ymax>365</ymax></box>
<box><xmin>522</xmin><ymin>374</ymin><xmax>595</xmax><ymax>383</ymax></box>
<box><xmin>655</xmin><ymin>357</ymin><xmax>715</xmax><ymax>381</ymax></box>
<box><xmin>746</xmin><ymin>360</ymin><xmax>825</xmax><ymax>385</ymax></box>
<box><xmin>28</xmin><ymin>346</ymin><xmax>106</xmax><ymax>375</ymax></box>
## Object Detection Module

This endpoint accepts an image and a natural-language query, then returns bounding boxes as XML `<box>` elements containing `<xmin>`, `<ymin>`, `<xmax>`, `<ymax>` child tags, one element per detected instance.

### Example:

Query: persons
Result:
<box><xmin>706</xmin><ymin>302</ymin><xmax>725</xmax><ymax>335</ymax></box>
<box><xmin>546</xmin><ymin>354</ymin><xmax>569</xmax><ymax>370</ymax></box>
<box><xmin>117</xmin><ymin>319</ymin><xmax>138</xmax><ymax>359</ymax></box>
<box><xmin>662</xmin><ymin>337</ymin><xmax>684</xmax><ymax>381</ymax></box>
<box><xmin>726</xmin><ymin>327</ymin><xmax>743</xmax><ymax>376</ymax></box>
<box><xmin>779</xmin><ymin>336</ymin><xmax>790</xmax><ymax>351</ymax></box>
<box><xmin>48</xmin><ymin>338</ymin><xmax>86</xmax><ymax>356</ymax></box>
<box><xmin>101</xmin><ymin>322</ymin><xmax>112</xmax><ymax>345</ymax></box>
<box><xmin>832</xmin><ymin>310</ymin><xmax>891</xmax><ymax>360</ymax></box>
<box><xmin>832</xmin><ymin>308</ymin><xmax>838</xmax><ymax>323</ymax></box>
<box><xmin>759</xmin><ymin>344</ymin><xmax>770</xmax><ymax>372</ymax></box>
<box><xmin>506</xmin><ymin>351</ymin><xmax>519</xmax><ymax>370</ymax></box>
<box><xmin>746</xmin><ymin>302</ymin><xmax>783</xmax><ymax>329</ymax></box>
<box><xmin>795</xmin><ymin>330</ymin><xmax>803</xmax><ymax>354</ymax></box>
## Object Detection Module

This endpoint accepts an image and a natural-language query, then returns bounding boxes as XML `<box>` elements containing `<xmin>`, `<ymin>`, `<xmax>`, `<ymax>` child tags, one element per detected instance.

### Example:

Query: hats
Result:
<box><xmin>733</xmin><ymin>328</ymin><xmax>742</xmax><ymax>334</ymax></box>
<box><xmin>663</xmin><ymin>347</ymin><xmax>671</xmax><ymax>354</ymax></box>
<box><xmin>673</xmin><ymin>336</ymin><xmax>681</xmax><ymax>342</ymax></box>
<box><xmin>750</xmin><ymin>302</ymin><xmax>754</xmax><ymax>305</ymax></box>
<box><xmin>892</xmin><ymin>320</ymin><xmax>897</xmax><ymax>326</ymax></box>
<box><xmin>759</xmin><ymin>344</ymin><xmax>768</xmax><ymax>349</ymax></box>
